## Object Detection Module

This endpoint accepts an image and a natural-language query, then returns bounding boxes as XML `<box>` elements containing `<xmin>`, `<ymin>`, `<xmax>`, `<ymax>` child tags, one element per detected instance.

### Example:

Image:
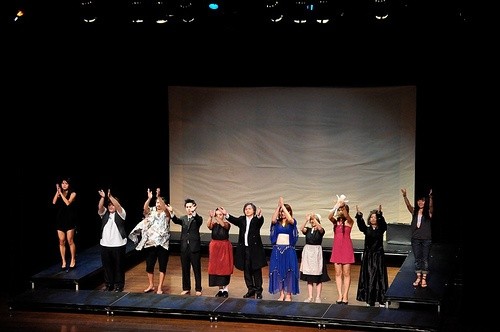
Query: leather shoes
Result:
<box><xmin>215</xmin><ymin>291</ymin><xmax>222</xmax><ymax>298</ymax></box>
<box><xmin>222</xmin><ymin>291</ymin><xmax>228</xmax><ymax>298</ymax></box>
<box><xmin>243</xmin><ymin>292</ymin><xmax>255</xmax><ymax>298</ymax></box>
<box><xmin>257</xmin><ymin>293</ymin><xmax>262</xmax><ymax>299</ymax></box>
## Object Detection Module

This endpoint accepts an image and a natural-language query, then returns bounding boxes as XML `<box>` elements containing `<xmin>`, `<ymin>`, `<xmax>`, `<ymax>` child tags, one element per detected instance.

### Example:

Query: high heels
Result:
<box><xmin>195</xmin><ymin>292</ymin><xmax>202</xmax><ymax>296</ymax></box>
<box><xmin>61</xmin><ymin>261</ymin><xmax>67</xmax><ymax>269</ymax></box>
<box><xmin>69</xmin><ymin>259</ymin><xmax>76</xmax><ymax>269</ymax></box>
<box><xmin>181</xmin><ymin>291</ymin><xmax>191</xmax><ymax>295</ymax></box>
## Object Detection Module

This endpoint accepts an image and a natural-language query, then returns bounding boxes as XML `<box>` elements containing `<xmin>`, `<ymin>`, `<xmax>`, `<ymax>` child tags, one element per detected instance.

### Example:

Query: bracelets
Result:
<box><xmin>340</xmin><ymin>205</ymin><xmax>345</xmax><ymax>208</ymax></box>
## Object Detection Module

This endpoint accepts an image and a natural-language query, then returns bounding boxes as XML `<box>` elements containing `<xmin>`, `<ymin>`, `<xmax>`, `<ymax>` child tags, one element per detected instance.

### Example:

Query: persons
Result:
<box><xmin>206</xmin><ymin>208</ymin><xmax>233</xmax><ymax>298</ymax></box>
<box><xmin>355</xmin><ymin>205</ymin><xmax>391</xmax><ymax>307</ymax></box>
<box><xmin>269</xmin><ymin>196</ymin><xmax>299</xmax><ymax>301</ymax></box>
<box><xmin>401</xmin><ymin>188</ymin><xmax>433</xmax><ymax>287</ymax></box>
<box><xmin>98</xmin><ymin>189</ymin><xmax>126</xmax><ymax>292</ymax></box>
<box><xmin>165</xmin><ymin>199</ymin><xmax>203</xmax><ymax>296</ymax></box>
<box><xmin>327</xmin><ymin>195</ymin><xmax>355</xmax><ymax>304</ymax></box>
<box><xmin>217</xmin><ymin>203</ymin><xmax>268</xmax><ymax>299</ymax></box>
<box><xmin>53</xmin><ymin>178</ymin><xmax>77</xmax><ymax>271</ymax></box>
<box><xmin>144</xmin><ymin>188</ymin><xmax>170</xmax><ymax>295</ymax></box>
<box><xmin>301</xmin><ymin>212</ymin><xmax>331</xmax><ymax>303</ymax></box>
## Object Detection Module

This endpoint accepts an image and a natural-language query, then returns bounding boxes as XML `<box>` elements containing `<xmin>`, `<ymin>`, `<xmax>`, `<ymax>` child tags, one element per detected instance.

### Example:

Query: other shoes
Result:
<box><xmin>413</xmin><ymin>274</ymin><xmax>420</xmax><ymax>286</ymax></box>
<box><xmin>314</xmin><ymin>298</ymin><xmax>321</xmax><ymax>303</ymax></box>
<box><xmin>336</xmin><ymin>300</ymin><xmax>342</xmax><ymax>304</ymax></box>
<box><xmin>342</xmin><ymin>302</ymin><xmax>348</xmax><ymax>305</ymax></box>
<box><xmin>278</xmin><ymin>294</ymin><xmax>284</xmax><ymax>301</ymax></box>
<box><xmin>104</xmin><ymin>286</ymin><xmax>114</xmax><ymax>292</ymax></box>
<box><xmin>285</xmin><ymin>294</ymin><xmax>292</xmax><ymax>301</ymax></box>
<box><xmin>303</xmin><ymin>297</ymin><xmax>313</xmax><ymax>303</ymax></box>
<box><xmin>421</xmin><ymin>275</ymin><xmax>428</xmax><ymax>287</ymax></box>
<box><xmin>115</xmin><ymin>287</ymin><xmax>124</xmax><ymax>293</ymax></box>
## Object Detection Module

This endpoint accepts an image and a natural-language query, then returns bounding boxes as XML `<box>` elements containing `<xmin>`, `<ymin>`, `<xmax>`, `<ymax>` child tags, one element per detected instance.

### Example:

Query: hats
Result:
<box><xmin>316</xmin><ymin>214</ymin><xmax>322</xmax><ymax>224</ymax></box>
